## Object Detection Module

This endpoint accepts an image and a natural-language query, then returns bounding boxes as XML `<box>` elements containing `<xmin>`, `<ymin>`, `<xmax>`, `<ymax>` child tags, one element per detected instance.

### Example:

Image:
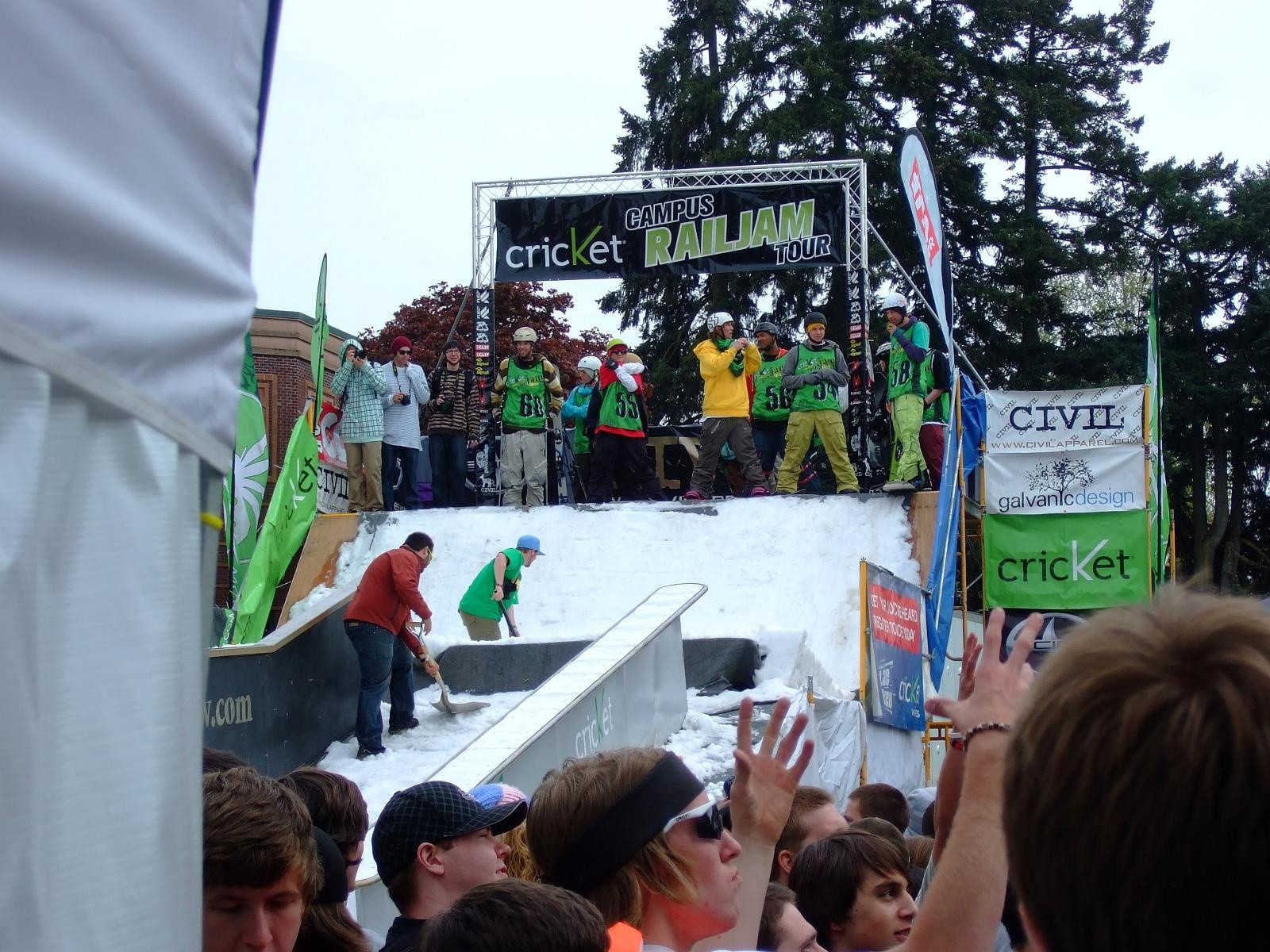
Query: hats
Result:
<box><xmin>303</xmin><ymin>824</ymin><xmax>348</xmax><ymax>903</ymax></box>
<box><xmin>517</xmin><ymin>534</ymin><xmax>546</xmax><ymax>556</ymax></box>
<box><xmin>469</xmin><ymin>784</ymin><xmax>532</xmax><ymax>823</ymax></box>
<box><xmin>371</xmin><ymin>780</ymin><xmax>528</xmax><ymax>868</ymax></box>
<box><xmin>390</xmin><ymin>336</ymin><xmax>413</xmax><ymax>355</ymax></box>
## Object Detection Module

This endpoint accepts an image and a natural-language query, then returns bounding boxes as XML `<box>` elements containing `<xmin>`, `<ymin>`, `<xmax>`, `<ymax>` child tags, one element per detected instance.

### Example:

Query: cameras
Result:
<box><xmin>401</xmin><ymin>395</ymin><xmax>411</xmax><ymax>406</ymax></box>
<box><xmin>439</xmin><ymin>401</ymin><xmax>453</xmax><ymax>412</ymax></box>
<box><xmin>352</xmin><ymin>350</ymin><xmax>366</xmax><ymax>362</ymax></box>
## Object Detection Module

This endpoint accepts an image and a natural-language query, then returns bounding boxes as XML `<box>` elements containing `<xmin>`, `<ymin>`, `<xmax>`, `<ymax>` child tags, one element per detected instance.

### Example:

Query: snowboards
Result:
<box><xmin>882</xmin><ymin>478</ymin><xmax>925</xmax><ymax>493</ymax></box>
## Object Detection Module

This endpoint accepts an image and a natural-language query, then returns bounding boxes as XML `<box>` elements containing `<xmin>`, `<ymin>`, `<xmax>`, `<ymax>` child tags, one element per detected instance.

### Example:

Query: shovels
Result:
<box><xmin>405</xmin><ymin>622</ymin><xmax>490</xmax><ymax>713</ymax></box>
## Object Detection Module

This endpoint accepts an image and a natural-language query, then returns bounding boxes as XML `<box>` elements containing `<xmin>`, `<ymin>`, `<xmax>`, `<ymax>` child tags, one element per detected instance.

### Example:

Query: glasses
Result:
<box><xmin>427</xmin><ymin>549</ymin><xmax>433</xmax><ymax>561</ymax></box>
<box><xmin>612</xmin><ymin>350</ymin><xmax>626</xmax><ymax>355</ymax></box>
<box><xmin>662</xmin><ymin>793</ymin><xmax>724</xmax><ymax>840</ymax></box>
<box><xmin>446</xmin><ymin>350</ymin><xmax>460</xmax><ymax>354</ymax></box>
<box><xmin>399</xmin><ymin>349</ymin><xmax>413</xmax><ymax>355</ymax></box>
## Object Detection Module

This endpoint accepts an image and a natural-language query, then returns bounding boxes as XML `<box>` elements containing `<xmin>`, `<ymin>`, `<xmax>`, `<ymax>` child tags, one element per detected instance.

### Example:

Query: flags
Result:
<box><xmin>898</xmin><ymin>127</ymin><xmax>954</xmax><ymax>370</ymax></box>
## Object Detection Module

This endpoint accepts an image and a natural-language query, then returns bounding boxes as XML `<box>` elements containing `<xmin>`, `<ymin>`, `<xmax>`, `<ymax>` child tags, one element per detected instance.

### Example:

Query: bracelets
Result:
<box><xmin>495</xmin><ymin>584</ymin><xmax>503</xmax><ymax>588</ymax></box>
<box><xmin>420</xmin><ymin>654</ymin><xmax>429</xmax><ymax>663</ymax></box>
<box><xmin>962</xmin><ymin>722</ymin><xmax>1011</xmax><ymax>751</ymax></box>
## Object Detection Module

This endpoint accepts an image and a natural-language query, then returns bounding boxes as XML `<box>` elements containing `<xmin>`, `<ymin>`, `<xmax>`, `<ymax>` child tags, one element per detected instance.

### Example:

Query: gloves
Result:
<box><xmin>606</xmin><ymin>360</ymin><xmax>618</xmax><ymax>370</ymax></box>
<box><xmin>804</xmin><ymin>370</ymin><xmax>823</xmax><ymax>383</ymax></box>
<box><xmin>642</xmin><ymin>368</ymin><xmax>650</xmax><ymax>382</ymax></box>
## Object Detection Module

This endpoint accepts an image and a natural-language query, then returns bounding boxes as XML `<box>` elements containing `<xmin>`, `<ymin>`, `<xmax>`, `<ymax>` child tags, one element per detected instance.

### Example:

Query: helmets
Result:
<box><xmin>514</xmin><ymin>327</ymin><xmax>537</xmax><ymax>343</ymax></box>
<box><xmin>882</xmin><ymin>293</ymin><xmax>907</xmax><ymax>313</ymax></box>
<box><xmin>607</xmin><ymin>338</ymin><xmax>628</xmax><ymax>351</ymax></box>
<box><xmin>578</xmin><ymin>356</ymin><xmax>602</xmax><ymax>373</ymax></box>
<box><xmin>707</xmin><ymin>312</ymin><xmax>733</xmax><ymax>332</ymax></box>
<box><xmin>804</xmin><ymin>312</ymin><xmax>827</xmax><ymax>330</ymax></box>
<box><xmin>753</xmin><ymin>322</ymin><xmax>778</xmax><ymax>338</ymax></box>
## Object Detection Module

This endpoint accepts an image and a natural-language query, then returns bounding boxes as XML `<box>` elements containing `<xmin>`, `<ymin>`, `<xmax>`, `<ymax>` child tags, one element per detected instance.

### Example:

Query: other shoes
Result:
<box><xmin>908</xmin><ymin>469</ymin><xmax>933</xmax><ymax>492</ymax></box>
<box><xmin>751</xmin><ymin>487</ymin><xmax>767</xmax><ymax>497</ymax></box>
<box><xmin>357</xmin><ymin>744</ymin><xmax>385</xmax><ymax>759</ymax></box>
<box><xmin>349</xmin><ymin>507</ymin><xmax>395</xmax><ymax>512</ymax></box>
<box><xmin>840</xmin><ymin>488</ymin><xmax>858</xmax><ymax>495</ymax></box>
<box><xmin>389</xmin><ymin>717</ymin><xmax>419</xmax><ymax>735</ymax></box>
<box><xmin>774</xmin><ymin>489</ymin><xmax>789</xmax><ymax>495</ymax></box>
<box><xmin>685</xmin><ymin>491</ymin><xmax>702</xmax><ymax>500</ymax></box>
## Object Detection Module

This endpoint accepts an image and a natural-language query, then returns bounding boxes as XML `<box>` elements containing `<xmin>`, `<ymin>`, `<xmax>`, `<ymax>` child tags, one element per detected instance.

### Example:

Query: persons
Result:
<box><xmin>489</xmin><ymin>327</ymin><xmax>564</xmax><ymax>506</ymax></box>
<box><xmin>419</xmin><ymin>340</ymin><xmax>481</xmax><ymax>508</ymax></box>
<box><xmin>202</xmin><ymin>583</ymin><xmax>1270</xmax><ymax>952</ymax></box>
<box><xmin>344</xmin><ymin>532</ymin><xmax>439</xmax><ymax>761</ymax></box>
<box><xmin>380</xmin><ymin>336</ymin><xmax>430</xmax><ymax>511</ymax></box>
<box><xmin>457</xmin><ymin>534</ymin><xmax>546</xmax><ymax>641</ymax></box>
<box><xmin>331</xmin><ymin>338</ymin><xmax>388</xmax><ymax>513</ymax></box>
<box><xmin>553</xmin><ymin>293</ymin><xmax>950</xmax><ymax>504</ymax></box>
